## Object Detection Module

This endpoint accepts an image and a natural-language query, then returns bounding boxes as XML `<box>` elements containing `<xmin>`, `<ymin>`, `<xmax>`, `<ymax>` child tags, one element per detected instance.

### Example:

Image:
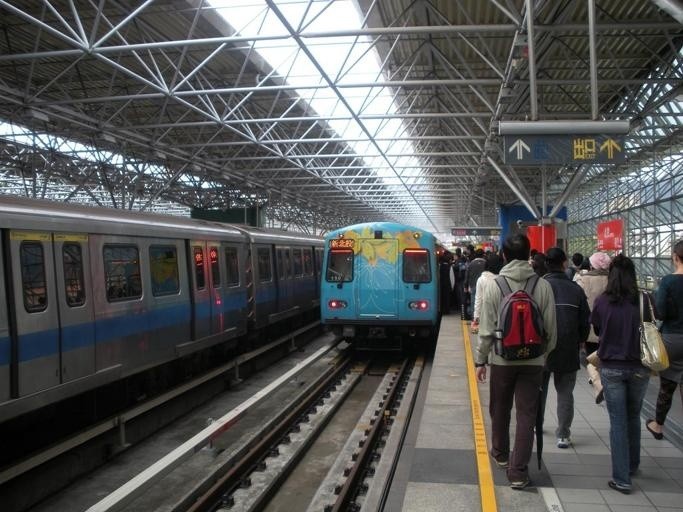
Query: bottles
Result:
<box><xmin>493</xmin><ymin>327</ymin><xmax>503</xmax><ymax>356</ymax></box>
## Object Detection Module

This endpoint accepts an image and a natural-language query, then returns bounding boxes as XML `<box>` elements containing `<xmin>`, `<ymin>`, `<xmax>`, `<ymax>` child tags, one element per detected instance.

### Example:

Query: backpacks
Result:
<box><xmin>494</xmin><ymin>275</ymin><xmax>547</xmax><ymax>360</ymax></box>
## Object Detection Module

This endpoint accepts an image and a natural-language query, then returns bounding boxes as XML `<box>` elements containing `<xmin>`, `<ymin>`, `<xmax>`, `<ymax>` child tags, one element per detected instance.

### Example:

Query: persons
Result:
<box><xmin>644</xmin><ymin>239</ymin><xmax>683</xmax><ymax>441</ymax></box>
<box><xmin>474</xmin><ymin>232</ymin><xmax>559</xmax><ymax>490</ymax></box>
<box><xmin>438</xmin><ymin>243</ymin><xmax>611</xmax><ymax>405</ymax></box>
<box><xmin>533</xmin><ymin>245</ymin><xmax>590</xmax><ymax>448</ymax></box>
<box><xmin>591</xmin><ymin>253</ymin><xmax>663</xmax><ymax>494</ymax></box>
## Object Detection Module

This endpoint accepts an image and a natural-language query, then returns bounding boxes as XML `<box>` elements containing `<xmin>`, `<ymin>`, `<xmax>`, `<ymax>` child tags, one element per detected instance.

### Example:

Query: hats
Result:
<box><xmin>589</xmin><ymin>252</ymin><xmax>611</xmax><ymax>270</ymax></box>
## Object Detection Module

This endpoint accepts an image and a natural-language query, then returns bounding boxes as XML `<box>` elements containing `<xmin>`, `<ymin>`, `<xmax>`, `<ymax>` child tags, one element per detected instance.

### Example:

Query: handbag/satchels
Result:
<box><xmin>638</xmin><ymin>322</ymin><xmax>669</xmax><ymax>372</ymax></box>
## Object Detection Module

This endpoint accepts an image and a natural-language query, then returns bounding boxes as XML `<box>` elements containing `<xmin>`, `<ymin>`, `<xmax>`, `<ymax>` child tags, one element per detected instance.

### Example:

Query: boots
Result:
<box><xmin>587</xmin><ymin>362</ymin><xmax>605</xmax><ymax>404</ymax></box>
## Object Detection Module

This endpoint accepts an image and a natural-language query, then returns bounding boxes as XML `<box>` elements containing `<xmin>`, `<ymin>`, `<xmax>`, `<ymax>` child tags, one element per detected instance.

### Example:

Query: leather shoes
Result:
<box><xmin>646</xmin><ymin>419</ymin><xmax>663</xmax><ymax>439</ymax></box>
<box><xmin>608</xmin><ymin>481</ymin><xmax>630</xmax><ymax>494</ymax></box>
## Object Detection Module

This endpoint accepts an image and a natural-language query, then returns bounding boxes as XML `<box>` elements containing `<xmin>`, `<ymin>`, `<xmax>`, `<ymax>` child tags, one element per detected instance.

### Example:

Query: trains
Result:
<box><xmin>0</xmin><ymin>190</ymin><xmax>327</xmax><ymax>446</ymax></box>
<box><xmin>317</xmin><ymin>221</ymin><xmax>448</xmax><ymax>367</ymax></box>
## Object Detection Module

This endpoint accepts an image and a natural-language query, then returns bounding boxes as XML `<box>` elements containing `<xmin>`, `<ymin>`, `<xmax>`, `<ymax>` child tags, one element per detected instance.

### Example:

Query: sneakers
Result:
<box><xmin>510</xmin><ymin>474</ymin><xmax>530</xmax><ymax>489</ymax></box>
<box><xmin>557</xmin><ymin>438</ymin><xmax>571</xmax><ymax>449</ymax></box>
<box><xmin>490</xmin><ymin>449</ymin><xmax>509</xmax><ymax>468</ymax></box>
